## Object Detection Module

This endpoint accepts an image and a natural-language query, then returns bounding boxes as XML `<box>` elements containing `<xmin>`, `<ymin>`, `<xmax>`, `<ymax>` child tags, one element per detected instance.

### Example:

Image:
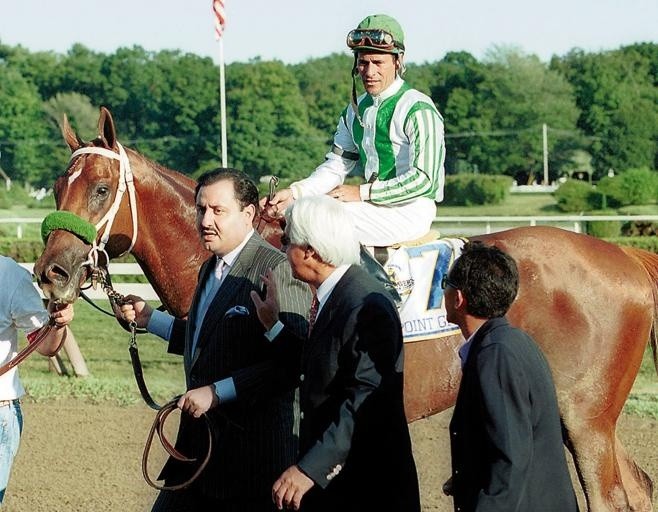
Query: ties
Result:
<box><xmin>192</xmin><ymin>259</ymin><xmax>227</xmax><ymax>357</ymax></box>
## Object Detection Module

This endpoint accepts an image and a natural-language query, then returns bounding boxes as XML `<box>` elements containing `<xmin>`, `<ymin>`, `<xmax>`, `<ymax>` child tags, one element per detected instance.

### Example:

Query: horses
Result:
<box><xmin>31</xmin><ymin>104</ymin><xmax>658</xmax><ymax>511</ymax></box>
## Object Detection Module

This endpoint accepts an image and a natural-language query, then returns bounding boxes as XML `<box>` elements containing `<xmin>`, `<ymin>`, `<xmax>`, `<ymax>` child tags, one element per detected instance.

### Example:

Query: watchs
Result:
<box><xmin>212</xmin><ymin>383</ymin><xmax>220</xmax><ymax>406</ymax></box>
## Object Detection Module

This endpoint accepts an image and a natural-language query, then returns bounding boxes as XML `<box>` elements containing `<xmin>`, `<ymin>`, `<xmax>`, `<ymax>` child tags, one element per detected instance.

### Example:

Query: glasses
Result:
<box><xmin>441</xmin><ymin>275</ymin><xmax>459</xmax><ymax>292</ymax></box>
<box><xmin>280</xmin><ymin>232</ymin><xmax>307</xmax><ymax>251</ymax></box>
<box><xmin>347</xmin><ymin>29</ymin><xmax>405</xmax><ymax>51</ymax></box>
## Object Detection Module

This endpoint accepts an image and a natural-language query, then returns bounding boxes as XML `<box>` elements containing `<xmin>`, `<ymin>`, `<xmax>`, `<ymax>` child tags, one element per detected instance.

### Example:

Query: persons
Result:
<box><xmin>120</xmin><ymin>168</ymin><xmax>314</xmax><ymax>512</ymax></box>
<box><xmin>251</xmin><ymin>195</ymin><xmax>421</xmax><ymax>512</ymax></box>
<box><xmin>258</xmin><ymin>14</ymin><xmax>446</xmax><ymax>246</ymax></box>
<box><xmin>442</xmin><ymin>242</ymin><xmax>579</xmax><ymax>512</ymax></box>
<box><xmin>0</xmin><ymin>254</ymin><xmax>74</xmax><ymax>512</ymax></box>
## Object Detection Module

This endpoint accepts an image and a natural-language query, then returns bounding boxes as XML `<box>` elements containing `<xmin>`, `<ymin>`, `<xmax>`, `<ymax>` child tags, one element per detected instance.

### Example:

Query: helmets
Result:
<box><xmin>346</xmin><ymin>14</ymin><xmax>405</xmax><ymax>54</ymax></box>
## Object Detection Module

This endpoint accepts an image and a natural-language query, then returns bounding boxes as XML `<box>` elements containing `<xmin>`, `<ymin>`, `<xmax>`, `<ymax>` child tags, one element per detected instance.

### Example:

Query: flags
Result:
<box><xmin>212</xmin><ymin>0</ymin><xmax>226</xmax><ymax>40</ymax></box>
